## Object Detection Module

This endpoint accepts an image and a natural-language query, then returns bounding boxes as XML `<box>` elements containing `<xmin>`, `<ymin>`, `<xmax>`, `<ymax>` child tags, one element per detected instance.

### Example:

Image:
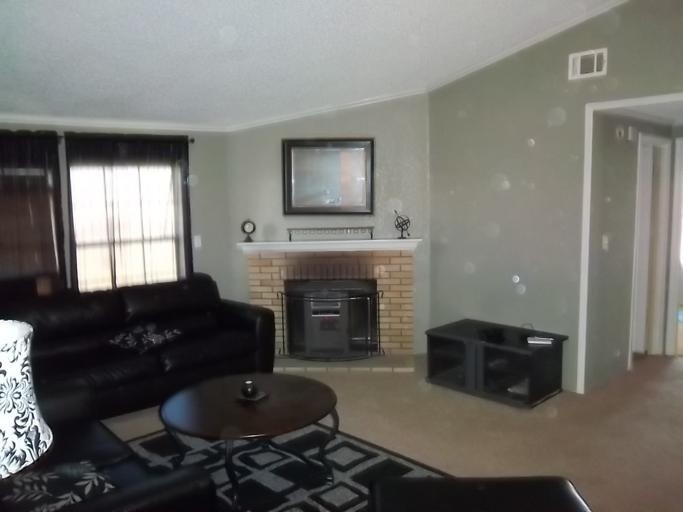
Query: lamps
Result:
<box><xmin>0</xmin><ymin>319</ymin><xmax>54</xmax><ymax>481</ymax></box>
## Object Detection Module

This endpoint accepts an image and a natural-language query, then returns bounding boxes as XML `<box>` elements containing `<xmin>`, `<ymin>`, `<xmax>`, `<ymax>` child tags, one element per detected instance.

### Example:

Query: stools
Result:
<box><xmin>368</xmin><ymin>476</ymin><xmax>592</xmax><ymax>512</ymax></box>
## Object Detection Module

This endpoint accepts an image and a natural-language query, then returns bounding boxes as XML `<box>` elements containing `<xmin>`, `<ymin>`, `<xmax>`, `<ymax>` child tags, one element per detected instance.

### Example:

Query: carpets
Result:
<box><xmin>124</xmin><ymin>422</ymin><xmax>455</xmax><ymax>512</ymax></box>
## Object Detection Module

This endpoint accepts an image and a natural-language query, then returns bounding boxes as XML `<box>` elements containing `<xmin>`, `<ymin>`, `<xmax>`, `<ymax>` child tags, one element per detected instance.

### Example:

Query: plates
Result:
<box><xmin>233</xmin><ymin>390</ymin><xmax>272</xmax><ymax>403</ymax></box>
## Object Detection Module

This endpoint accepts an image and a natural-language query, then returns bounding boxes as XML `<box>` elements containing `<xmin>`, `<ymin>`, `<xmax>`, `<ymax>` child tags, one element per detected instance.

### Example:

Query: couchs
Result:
<box><xmin>0</xmin><ymin>374</ymin><xmax>216</xmax><ymax>511</ymax></box>
<box><xmin>0</xmin><ymin>273</ymin><xmax>276</xmax><ymax>420</ymax></box>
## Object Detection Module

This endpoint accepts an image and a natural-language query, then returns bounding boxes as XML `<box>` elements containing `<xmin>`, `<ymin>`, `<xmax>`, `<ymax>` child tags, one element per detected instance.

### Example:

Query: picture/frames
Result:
<box><xmin>282</xmin><ymin>138</ymin><xmax>375</xmax><ymax>215</ymax></box>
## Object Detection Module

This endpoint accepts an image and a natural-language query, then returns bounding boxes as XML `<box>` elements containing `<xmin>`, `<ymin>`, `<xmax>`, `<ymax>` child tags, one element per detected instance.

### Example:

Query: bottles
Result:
<box><xmin>242</xmin><ymin>379</ymin><xmax>257</xmax><ymax>397</ymax></box>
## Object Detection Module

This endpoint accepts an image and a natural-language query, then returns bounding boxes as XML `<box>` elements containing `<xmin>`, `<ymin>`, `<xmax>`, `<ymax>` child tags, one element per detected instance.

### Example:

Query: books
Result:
<box><xmin>526</xmin><ymin>335</ymin><xmax>554</xmax><ymax>345</ymax></box>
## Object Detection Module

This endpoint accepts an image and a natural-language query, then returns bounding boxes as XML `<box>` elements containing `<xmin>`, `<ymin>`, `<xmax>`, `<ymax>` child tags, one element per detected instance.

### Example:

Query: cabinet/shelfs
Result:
<box><xmin>425</xmin><ymin>317</ymin><xmax>569</xmax><ymax>411</ymax></box>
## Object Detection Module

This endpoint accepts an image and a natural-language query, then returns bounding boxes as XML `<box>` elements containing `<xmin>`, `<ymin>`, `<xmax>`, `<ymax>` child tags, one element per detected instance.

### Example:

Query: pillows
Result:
<box><xmin>1</xmin><ymin>458</ymin><xmax>121</xmax><ymax>512</ymax></box>
<box><xmin>106</xmin><ymin>320</ymin><xmax>184</xmax><ymax>358</ymax></box>
<box><xmin>0</xmin><ymin>471</ymin><xmax>87</xmax><ymax>511</ymax></box>
